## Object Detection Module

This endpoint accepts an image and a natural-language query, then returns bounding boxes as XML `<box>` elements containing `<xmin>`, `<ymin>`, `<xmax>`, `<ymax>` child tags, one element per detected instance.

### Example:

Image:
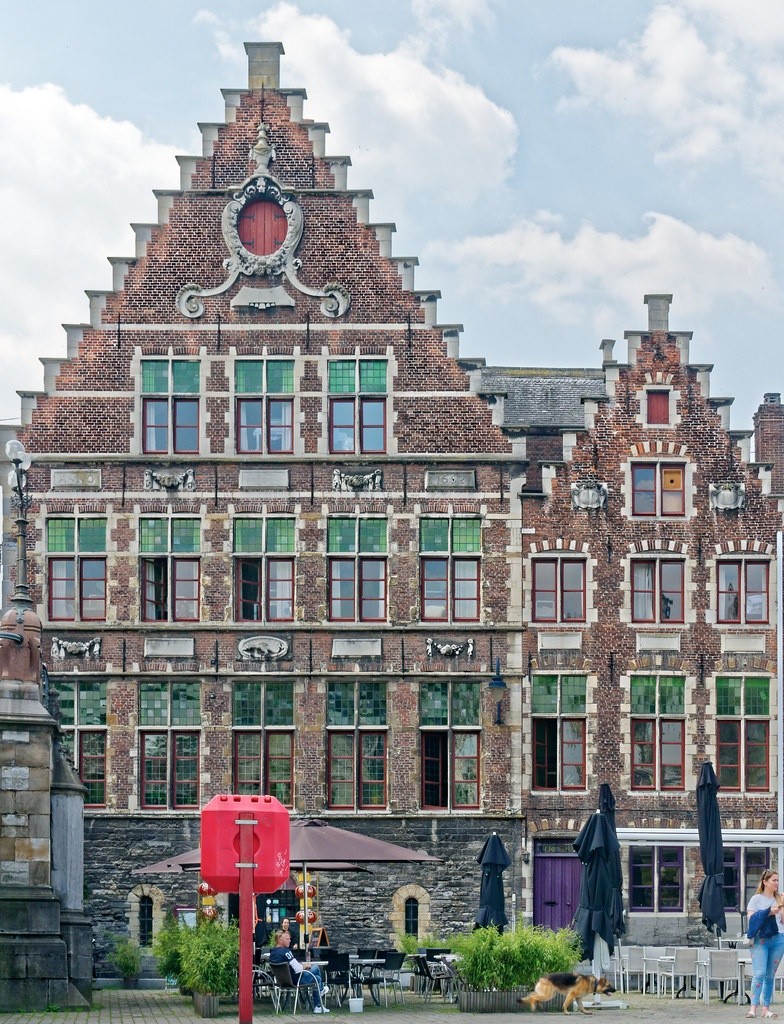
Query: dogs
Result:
<box><xmin>516</xmin><ymin>973</ymin><xmax>617</xmax><ymax>1016</ymax></box>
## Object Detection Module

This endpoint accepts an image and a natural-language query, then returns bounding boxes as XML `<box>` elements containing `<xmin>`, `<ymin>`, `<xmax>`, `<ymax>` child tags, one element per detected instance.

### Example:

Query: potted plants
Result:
<box><xmin>394</xmin><ymin>915</ymin><xmax>582</xmax><ymax>1012</ymax></box>
<box><xmin>151</xmin><ymin>911</ymin><xmax>241</xmax><ymax>1019</ymax></box>
<box><xmin>108</xmin><ymin>934</ymin><xmax>144</xmax><ymax>989</ymax></box>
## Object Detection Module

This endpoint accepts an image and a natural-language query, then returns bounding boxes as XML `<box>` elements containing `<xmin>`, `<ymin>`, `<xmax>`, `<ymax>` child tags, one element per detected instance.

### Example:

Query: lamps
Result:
<box><xmin>521</xmin><ymin>851</ymin><xmax>530</xmax><ymax>865</ymax></box>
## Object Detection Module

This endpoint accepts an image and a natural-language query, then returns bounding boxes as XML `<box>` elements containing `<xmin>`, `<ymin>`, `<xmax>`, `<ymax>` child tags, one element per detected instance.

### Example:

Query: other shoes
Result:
<box><xmin>746</xmin><ymin>1012</ymin><xmax>756</xmax><ymax>1018</ymax></box>
<box><xmin>762</xmin><ymin>1012</ymin><xmax>777</xmax><ymax>1019</ymax></box>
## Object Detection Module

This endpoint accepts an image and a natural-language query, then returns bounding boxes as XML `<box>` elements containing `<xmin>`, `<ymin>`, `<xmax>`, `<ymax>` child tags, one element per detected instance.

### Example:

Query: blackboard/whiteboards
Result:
<box><xmin>309</xmin><ymin>928</ymin><xmax>330</xmax><ymax>947</ymax></box>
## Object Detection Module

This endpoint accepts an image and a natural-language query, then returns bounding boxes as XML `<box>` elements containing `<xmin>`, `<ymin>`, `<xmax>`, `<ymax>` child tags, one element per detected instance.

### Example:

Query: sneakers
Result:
<box><xmin>321</xmin><ymin>985</ymin><xmax>329</xmax><ymax>997</ymax></box>
<box><xmin>313</xmin><ymin>1005</ymin><xmax>330</xmax><ymax>1014</ymax></box>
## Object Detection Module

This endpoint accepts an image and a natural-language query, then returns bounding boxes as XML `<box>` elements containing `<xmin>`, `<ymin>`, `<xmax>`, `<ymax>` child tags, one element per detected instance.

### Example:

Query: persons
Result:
<box><xmin>281</xmin><ymin>918</ymin><xmax>298</xmax><ymax>949</ymax></box>
<box><xmin>745</xmin><ymin>871</ymin><xmax>784</xmax><ymax>1018</ymax></box>
<box><xmin>269</xmin><ymin>931</ymin><xmax>329</xmax><ymax>1014</ymax></box>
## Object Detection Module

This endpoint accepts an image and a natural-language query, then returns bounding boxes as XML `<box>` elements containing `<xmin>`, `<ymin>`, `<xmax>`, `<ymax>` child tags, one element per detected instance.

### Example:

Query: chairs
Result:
<box><xmin>612</xmin><ymin>946</ymin><xmax>784</xmax><ymax>1006</ymax></box>
<box><xmin>251</xmin><ymin>947</ymin><xmax>468</xmax><ymax>1016</ymax></box>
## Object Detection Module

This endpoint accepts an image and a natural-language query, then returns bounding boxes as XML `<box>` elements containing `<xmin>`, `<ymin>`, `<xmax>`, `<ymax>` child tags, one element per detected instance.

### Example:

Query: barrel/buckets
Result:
<box><xmin>348</xmin><ymin>997</ymin><xmax>364</xmax><ymax>1012</ymax></box>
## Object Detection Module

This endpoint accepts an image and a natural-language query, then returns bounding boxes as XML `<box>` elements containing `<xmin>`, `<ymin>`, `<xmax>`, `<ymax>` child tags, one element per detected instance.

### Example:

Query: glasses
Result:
<box><xmin>763</xmin><ymin>868</ymin><xmax>771</xmax><ymax>878</ymax></box>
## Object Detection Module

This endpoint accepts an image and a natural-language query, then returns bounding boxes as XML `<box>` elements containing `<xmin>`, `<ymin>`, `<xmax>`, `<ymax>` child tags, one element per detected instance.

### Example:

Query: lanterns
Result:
<box><xmin>295</xmin><ymin>884</ymin><xmax>316</xmax><ymax>899</ymax></box>
<box><xmin>296</xmin><ymin>909</ymin><xmax>317</xmax><ymax>925</ymax></box>
<box><xmin>199</xmin><ymin>881</ymin><xmax>218</xmax><ymax>920</ymax></box>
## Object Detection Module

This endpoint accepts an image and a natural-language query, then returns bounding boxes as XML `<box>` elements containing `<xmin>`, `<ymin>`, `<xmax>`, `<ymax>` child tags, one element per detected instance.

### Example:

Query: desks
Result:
<box><xmin>723</xmin><ymin>958</ymin><xmax>753</xmax><ymax>1005</ymax></box>
<box><xmin>660</xmin><ymin>956</ymin><xmax>704</xmax><ymax>998</ymax></box>
<box><xmin>297</xmin><ymin>959</ymin><xmax>386</xmax><ymax>1007</ymax></box>
<box><xmin>406</xmin><ymin>954</ymin><xmax>464</xmax><ymax>1003</ymax></box>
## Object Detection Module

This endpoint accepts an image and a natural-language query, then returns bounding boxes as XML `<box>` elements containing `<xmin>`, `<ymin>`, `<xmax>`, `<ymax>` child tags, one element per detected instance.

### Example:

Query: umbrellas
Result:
<box><xmin>176</xmin><ymin>820</ymin><xmax>443</xmax><ymax>959</ymax></box>
<box><xmin>570</xmin><ymin>809</ymin><xmax>620</xmax><ymax>1003</ymax></box>
<box><xmin>472</xmin><ymin>832</ymin><xmax>511</xmax><ymax>935</ymax></box>
<box><xmin>695</xmin><ymin>762</ymin><xmax>726</xmax><ymax>1000</ymax></box>
<box><xmin>133</xmin><ymin>848</ymin><xmax>373</xmax><ymax>956</ymax></box>
<box><xmin>598</xmin><ymin>780</ymin><xmax>626</xmax><ymax>991</ymax></box>
